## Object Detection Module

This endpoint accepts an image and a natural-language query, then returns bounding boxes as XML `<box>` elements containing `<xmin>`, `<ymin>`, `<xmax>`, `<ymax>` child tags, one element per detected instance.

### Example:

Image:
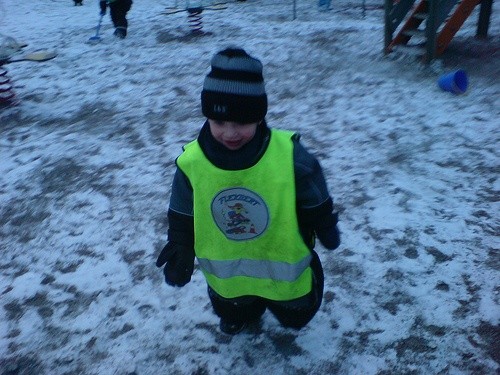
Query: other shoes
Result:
<box><xmin>219</xmin><ymin>317</ymin><xmax>246</xmax><ymax>334</ymax></box>
<box><xmin>113</xmin><ymin>29</ymin><xmax>127</xmax><ymax>38</ymax></box>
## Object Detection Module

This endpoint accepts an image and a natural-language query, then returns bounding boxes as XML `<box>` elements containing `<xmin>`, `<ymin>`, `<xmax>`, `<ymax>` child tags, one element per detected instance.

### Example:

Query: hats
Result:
<box><xmin>201</xmin><ymin>49</ymin><xmax>267</xmax><ymax>121</ymax></box>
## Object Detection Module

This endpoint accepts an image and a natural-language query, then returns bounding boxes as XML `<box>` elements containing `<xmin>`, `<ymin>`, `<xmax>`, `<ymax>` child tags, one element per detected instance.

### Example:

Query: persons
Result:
<box><xmin>73</xmin><ymin>0</ymin><xmax>133</xmax><ymax>39</ymax></box>
<box><xmin>156</xmin><ymin>48</ymin><xmax>342</xmax><ymax>336</ymax></box>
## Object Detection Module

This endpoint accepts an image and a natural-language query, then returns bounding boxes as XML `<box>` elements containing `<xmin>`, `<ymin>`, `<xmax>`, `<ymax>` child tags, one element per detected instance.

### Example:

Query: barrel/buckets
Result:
<box><xmin>438</xmin><ymin>69</ymin><xmax>469</xmax><ymax>94</ymax></box>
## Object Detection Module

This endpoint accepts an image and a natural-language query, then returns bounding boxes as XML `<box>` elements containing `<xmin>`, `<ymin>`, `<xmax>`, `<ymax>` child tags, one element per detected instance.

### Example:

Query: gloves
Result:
<box><xmin>156</xmin><ymin>241</ymin><xmax>195</xmax><ymax>287</ymax></box>
<box><xmin>315</xmin><ymin>213</ymin><xmax>341</xmax><ymax>250</ymax></box>
